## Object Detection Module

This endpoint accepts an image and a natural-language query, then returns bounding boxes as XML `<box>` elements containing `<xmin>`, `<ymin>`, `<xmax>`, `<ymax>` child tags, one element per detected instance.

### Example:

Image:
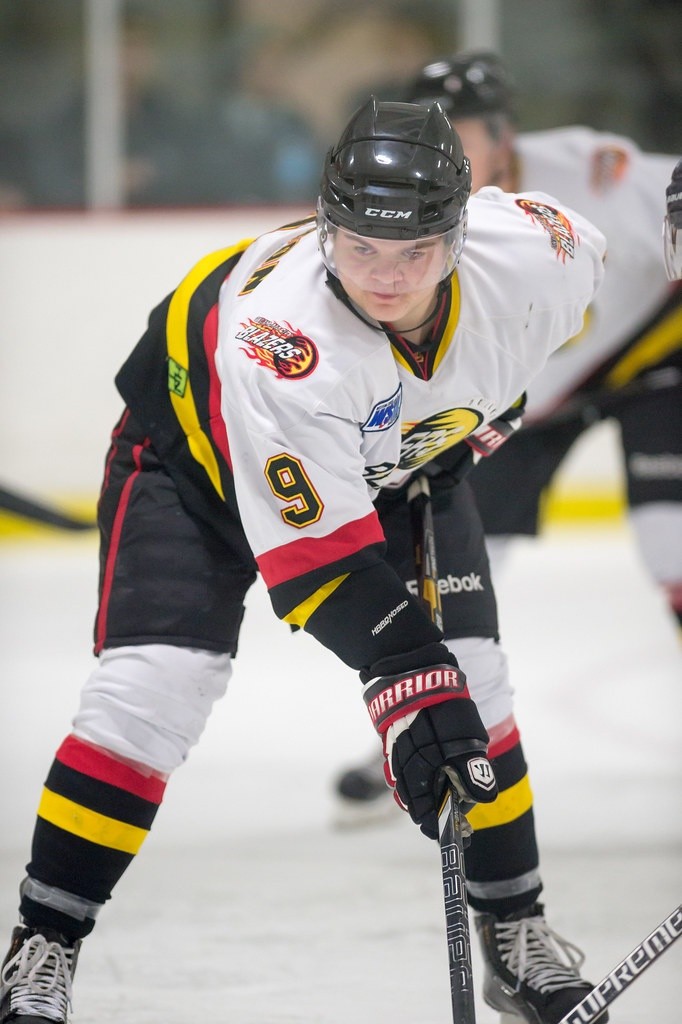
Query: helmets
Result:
<box><xmin>409</xmin><ymin>49</ymin><xmax>511</xmax><ymax>116</ymax></box>
<box><xmin>665</xmin><ymin>157</ymin><xmax>682</xmax><ymax>229</ymax></box>
<box><xmin>316</xmin><ymin>95</ymin><xmax>472</xmax><ymax>241</ymax></box>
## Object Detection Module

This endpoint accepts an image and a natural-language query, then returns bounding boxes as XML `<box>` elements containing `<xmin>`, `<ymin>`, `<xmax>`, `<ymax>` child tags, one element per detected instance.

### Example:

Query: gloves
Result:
<box><xmin>400</xmin><ymin>418</ymin><xmax>522</xmax><ymax>494</ymax></box>
<box><xmin>358</xmin><ymin>640</ymin><xmax>499</xmax><ymax>840</ymax></box>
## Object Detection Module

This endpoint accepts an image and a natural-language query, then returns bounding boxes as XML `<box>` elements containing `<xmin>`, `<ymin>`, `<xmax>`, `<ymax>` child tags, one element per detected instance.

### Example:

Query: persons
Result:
<box><xmin>0</xmin><ymin>94</ymin><xmax>611</xmax><ymax>1024</ymax></box>
<box><xmin>662</xmin><ymin>158</ymin><xmax>682</xmax><ymax>281</ymax></box>
<box><xmin>334</xmin><ymin>52</ymin><xmax>682</xmax><ymax>828</ymax></box>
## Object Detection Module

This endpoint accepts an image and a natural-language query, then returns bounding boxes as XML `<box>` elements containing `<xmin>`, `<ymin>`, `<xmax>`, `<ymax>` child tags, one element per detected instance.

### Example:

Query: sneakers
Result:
<box><xmin>0</xmin><ymin>914</ymin><xmax>84</xmax><ymax>1024</ymax></box>
<box><xmin>332</xmin><ymin>746</ymin><xmax>390</xmax><ymax>820</ymax></box>
<box><xmin>473</xmin><ymin>900</ymin><xmax>609</xmax><ymax>1024</ymax></box>
